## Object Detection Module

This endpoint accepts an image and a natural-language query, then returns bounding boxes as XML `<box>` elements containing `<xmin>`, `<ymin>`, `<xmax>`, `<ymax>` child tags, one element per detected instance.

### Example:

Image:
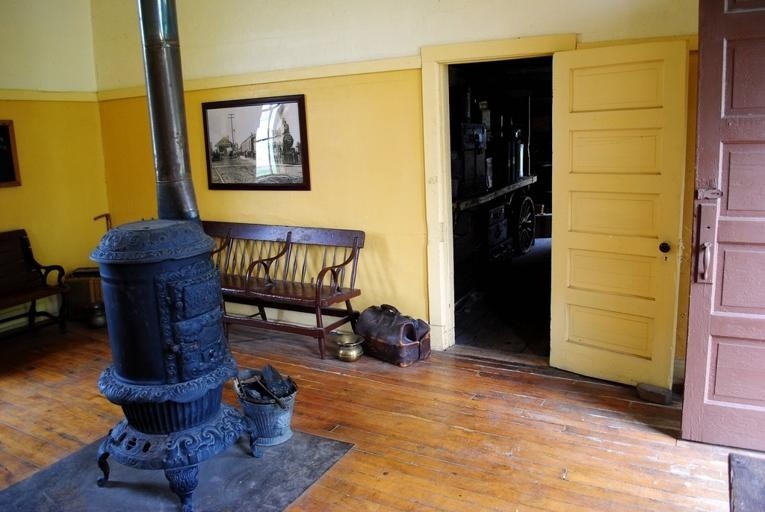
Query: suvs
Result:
<box><xmin>233</xmin><ymin>370</ymin><xmax>298</xmax><ymax>448</ymax></box>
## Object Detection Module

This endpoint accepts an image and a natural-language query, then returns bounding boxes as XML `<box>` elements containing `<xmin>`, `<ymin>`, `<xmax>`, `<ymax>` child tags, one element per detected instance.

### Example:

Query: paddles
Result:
<box><xmin>727</xmin><ymin>453</ymin><xmax>765</xmax><ymax>511</ymax></box>
<box><xmin>1</xmin><ymin>430</ymin><xmax>356</xmax><ymax>510</ymax></box>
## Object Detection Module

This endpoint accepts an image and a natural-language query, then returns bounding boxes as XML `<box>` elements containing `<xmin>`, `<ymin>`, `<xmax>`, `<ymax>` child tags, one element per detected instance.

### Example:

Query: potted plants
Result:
<box><xmin>0</xmin><ymin>119</ymin><xmax>22</xmax><ymax>188</ymax></box>
<box><xmin>201</xmin><ymin>94</ymin><xmax>311</xmax><ymax>191</ymax></box>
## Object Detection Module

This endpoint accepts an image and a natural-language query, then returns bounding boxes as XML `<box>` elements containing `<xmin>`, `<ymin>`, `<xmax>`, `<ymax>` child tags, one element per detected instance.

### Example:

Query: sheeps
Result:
<box><xmin>88</xmin><ymin>211</ymin><xmax>111</xmax><ymax>235</ymax></box>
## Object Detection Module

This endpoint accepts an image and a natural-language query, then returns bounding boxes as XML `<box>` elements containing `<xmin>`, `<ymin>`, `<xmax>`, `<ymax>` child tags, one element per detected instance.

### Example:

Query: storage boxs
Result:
<box><xmin>198</xmin><ymin>221</ymin><xmax>366</xmax><ymax>360</ymax></box>
<box><xmin>1</xmin><ymin>229</ymin><xmax>70</xmax><ymax>344</ymax></box>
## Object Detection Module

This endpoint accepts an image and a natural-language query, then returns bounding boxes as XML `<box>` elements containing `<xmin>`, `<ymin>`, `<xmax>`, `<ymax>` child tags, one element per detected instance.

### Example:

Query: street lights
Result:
<box><xmin>356</xmin><ymin>304</ymin><xmax>431</xmax><ymax>367</ymax></box>
